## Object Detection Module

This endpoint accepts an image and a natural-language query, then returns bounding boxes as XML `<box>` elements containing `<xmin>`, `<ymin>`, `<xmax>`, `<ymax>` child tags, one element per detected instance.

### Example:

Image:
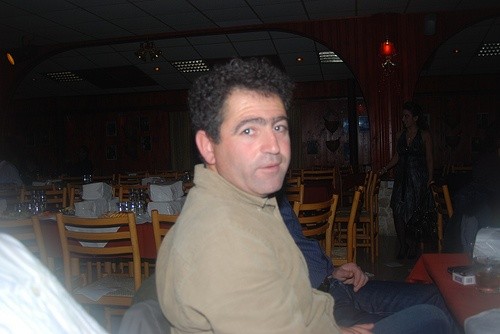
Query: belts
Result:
<box><xmin>317</xmin><ymin>276</ymin><xmax>335</xmax><ymax>291</ymax></box>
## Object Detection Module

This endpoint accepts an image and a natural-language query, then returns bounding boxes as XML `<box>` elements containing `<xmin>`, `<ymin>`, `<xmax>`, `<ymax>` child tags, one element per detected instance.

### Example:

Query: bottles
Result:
<box><xmin>82</xmin><ymin>174</ymin><xmax>93</xmax><ymax>185</ymax></box>
<box><xmin>118</xmin><ymin>187</ymin><xmax>146</xmax><ymax>216</ymax></box>
<box><xmin>16</xmin><ymin>190</ymin><xmax>48</xmax><ymax>216</ymax></box>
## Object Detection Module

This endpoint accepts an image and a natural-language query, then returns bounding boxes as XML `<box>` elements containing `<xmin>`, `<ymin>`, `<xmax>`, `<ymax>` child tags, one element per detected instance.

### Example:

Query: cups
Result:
<box><xmin>472</xmin><ymin>255</ymin><xmax>500</xmax><ymax>294</ymax></box>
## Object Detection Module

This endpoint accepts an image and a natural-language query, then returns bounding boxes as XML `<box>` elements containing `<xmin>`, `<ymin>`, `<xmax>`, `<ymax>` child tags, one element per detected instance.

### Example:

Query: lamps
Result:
<box><xmin>377</xmin><ymin>41</ymin><xmax>399</xmax><ymax>68</ymax></box>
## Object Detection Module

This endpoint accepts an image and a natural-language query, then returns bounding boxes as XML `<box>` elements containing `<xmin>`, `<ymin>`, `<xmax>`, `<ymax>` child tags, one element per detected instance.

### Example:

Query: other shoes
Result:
<box><xmin>385</xmin><ymin>261</ymin><xmax>404</xmax><ymax>268</ymax></box>
<box><xmin>408</xmin><ymin>267</ymin><xmax>412</xmax><ymax>272</ymax></box>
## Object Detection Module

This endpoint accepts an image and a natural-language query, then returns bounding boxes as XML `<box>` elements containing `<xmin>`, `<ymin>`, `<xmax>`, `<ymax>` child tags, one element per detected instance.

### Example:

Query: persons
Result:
<box><xmin>155</xmin><ymin>57</ymin><xmax>462</xmax><ymax>334</ymax></box>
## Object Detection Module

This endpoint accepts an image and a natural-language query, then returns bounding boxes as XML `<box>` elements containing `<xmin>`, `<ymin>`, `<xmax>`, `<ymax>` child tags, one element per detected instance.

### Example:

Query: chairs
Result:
<box><xmin>1</xmin><ymin>169</ymin><xmax>383</xmax><ymax>333</ymax></box>
<box><xmin>430</xmin><ymin>180</ymin><xmax>454</xmax><ymax>253</ymax></box>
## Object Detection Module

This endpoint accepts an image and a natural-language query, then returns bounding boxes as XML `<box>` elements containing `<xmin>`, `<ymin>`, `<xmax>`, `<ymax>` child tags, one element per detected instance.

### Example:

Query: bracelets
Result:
<box><xmin>383</xmin><ymin>166</ymin><xmax>387</xmax><ymax>173</ymax></box>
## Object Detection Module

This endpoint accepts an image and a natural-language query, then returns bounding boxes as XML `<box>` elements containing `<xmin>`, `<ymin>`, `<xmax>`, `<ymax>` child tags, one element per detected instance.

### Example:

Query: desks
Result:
<box><xmin>405</xmin><ymin>253</ymin><xmax>499</xmax><ymax>334</ymax></box>
<box><xmin>0</xmin><ymin>219</ymin><xmax>175</xmax><ymax>259</ymax></box>
